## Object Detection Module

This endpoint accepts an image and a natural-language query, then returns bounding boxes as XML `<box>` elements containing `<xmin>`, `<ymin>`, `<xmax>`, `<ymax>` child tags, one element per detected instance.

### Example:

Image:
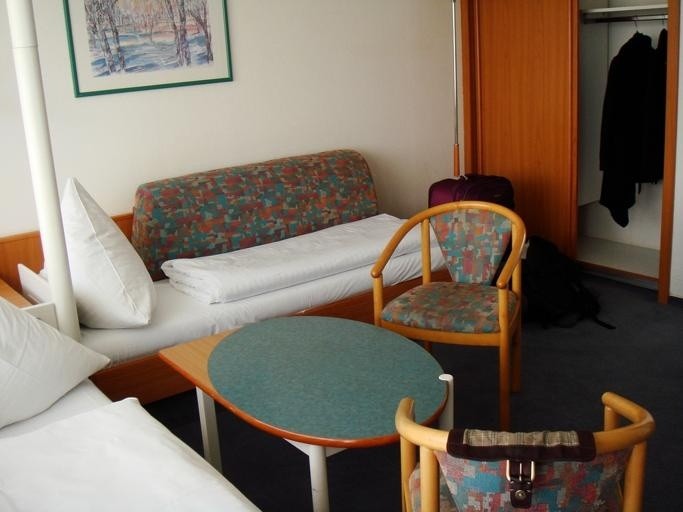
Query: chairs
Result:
<box><xmin>393</xmin><ymin>390</ymin><xmax>655</xmax><ymax>512</ymax></box>
<box><xmin>371</xmin><ymin>201</ymin><xmax>527</xmax><ymax>433</ymax></box>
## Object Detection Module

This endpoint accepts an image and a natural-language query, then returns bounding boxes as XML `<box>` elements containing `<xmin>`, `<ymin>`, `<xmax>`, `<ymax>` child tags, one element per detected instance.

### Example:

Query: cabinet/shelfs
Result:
<box><xmin>457</xmin><ymin>0</ymin><xmax>682</xmax><ymax>306</ymax></box>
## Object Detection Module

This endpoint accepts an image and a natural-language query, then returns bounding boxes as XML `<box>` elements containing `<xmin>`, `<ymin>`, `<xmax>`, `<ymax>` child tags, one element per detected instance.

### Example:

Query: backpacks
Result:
<box><xmin>491</xmin><ymin>232</ymin><xmax>617</xmax><ymax>330</ymax></box>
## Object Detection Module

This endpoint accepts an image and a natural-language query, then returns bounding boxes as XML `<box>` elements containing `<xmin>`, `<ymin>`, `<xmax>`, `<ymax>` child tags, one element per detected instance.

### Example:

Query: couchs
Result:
<box><xmin>0</xmin><ymin>204</ymin><xmax>452</xmax><ymax>403</ymax></box>
<box><xmin>0</xmin><ymin>302</ymin><xmax>268</xmax><ymax>512</ymax></box>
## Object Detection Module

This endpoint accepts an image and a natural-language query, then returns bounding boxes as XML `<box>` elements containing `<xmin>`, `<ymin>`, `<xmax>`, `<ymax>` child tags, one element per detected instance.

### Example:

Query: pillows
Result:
<box><xmin>35</xmin><ymin>177</ymin><xmax>155</xmax><ymax>328</ymax></box>
<box><xmin>132</xmin><ymin>151</ymin><xmax>379</xmax><ymax>281</ymax></box>
<box><xmin>0</xmin><ymin>297</ymin><xmax>110</xmax><ymax>430</ymax></box>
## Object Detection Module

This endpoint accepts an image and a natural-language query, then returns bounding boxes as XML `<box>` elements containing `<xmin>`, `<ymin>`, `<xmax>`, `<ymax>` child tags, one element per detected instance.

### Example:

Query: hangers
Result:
<box><xmin>628</xmin><ymin>12</ymin><xmax>667</xmax><ymax>41</ymax></box>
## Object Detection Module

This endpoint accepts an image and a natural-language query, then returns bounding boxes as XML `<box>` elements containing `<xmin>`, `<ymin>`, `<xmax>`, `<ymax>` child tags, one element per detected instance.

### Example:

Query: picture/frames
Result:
<box><xmin>62</xmin><ymin>0</ymin><xmax>234</xmax><ymax>97</ymax></box>
